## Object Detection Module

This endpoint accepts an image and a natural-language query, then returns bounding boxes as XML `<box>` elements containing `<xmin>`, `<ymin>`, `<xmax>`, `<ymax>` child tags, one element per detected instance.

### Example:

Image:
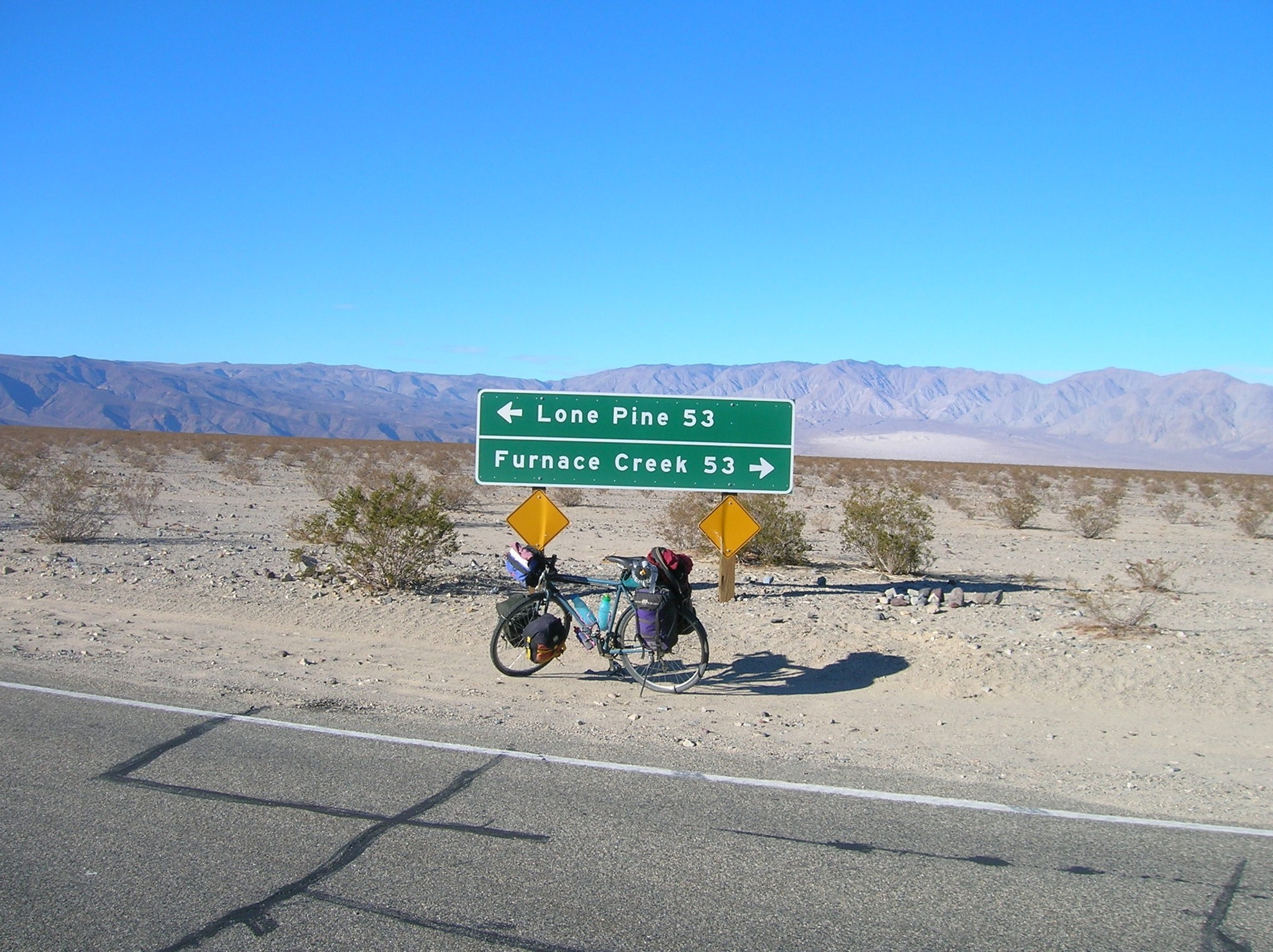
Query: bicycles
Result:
<box><xmin>488</xmin><ymin>549</ymin><xmax>710</xmax><ymax>700</ymax></box>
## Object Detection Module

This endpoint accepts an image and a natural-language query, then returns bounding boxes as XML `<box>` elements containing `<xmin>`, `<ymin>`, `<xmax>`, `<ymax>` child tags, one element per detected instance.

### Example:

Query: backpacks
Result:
<box><xmin>633</xmin><ymin>584</ymin><xmax>679</xmax><ymax>660</ymax></box>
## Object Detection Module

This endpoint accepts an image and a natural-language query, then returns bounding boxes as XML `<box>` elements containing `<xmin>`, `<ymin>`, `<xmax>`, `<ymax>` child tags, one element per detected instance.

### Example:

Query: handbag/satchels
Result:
<box><xmin>496</xmin><ymin>594</ymin><xmax>541</xmax><ymax>648</ymax></box>
<box><xmin>523</xmin><ymin>613</ymin><xmax>568</xmax><ymax>664</ymax></box>
<box><xmin>647</xmin><ymin>546</ymin><xmax>696</xmax><ymax>635</ymax></box>
<box><xmin>502</xmin><ymin>542</ymin><xmax>551</xmax><ymax>588</ymax></box>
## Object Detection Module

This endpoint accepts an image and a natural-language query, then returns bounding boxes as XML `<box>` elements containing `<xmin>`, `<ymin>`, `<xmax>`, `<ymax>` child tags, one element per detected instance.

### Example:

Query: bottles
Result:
<box><xmin>594</xmin><ymin>593</ymin><xmax>612</xmax><ymax>631</ymax></box>
<box><xmin>573</xmin><ymin>626</ymin><xmax>595</xmax><ymax>650</ymax></box>
<box><xmin>570</xmin><ymin>592</ymin><xmax>597</xmax><ymax>627</ymax></box>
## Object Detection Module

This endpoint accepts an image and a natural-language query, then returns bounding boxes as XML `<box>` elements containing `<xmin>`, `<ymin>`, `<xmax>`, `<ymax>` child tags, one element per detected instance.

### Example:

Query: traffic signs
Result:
<box><xmin>474</xmin><ymin>434</ymin><xmax>794</xmax><ymax>495</ymax></box>
<box><xmin>475</xmin><ymin>387</ymin><xmax>795</xmax><ymax>448</ymax></box>
